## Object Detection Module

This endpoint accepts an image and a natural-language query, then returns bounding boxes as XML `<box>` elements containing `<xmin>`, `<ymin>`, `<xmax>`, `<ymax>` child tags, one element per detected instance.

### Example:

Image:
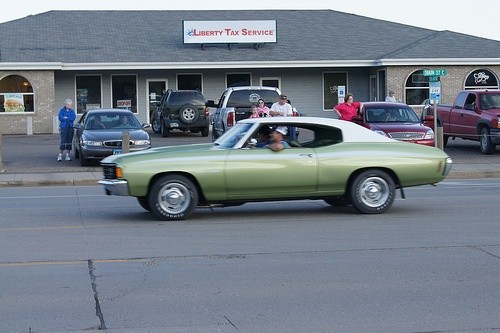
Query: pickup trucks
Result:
<box><xmin>423</xmin><ymin>88</ymin><xmax>500</xmax><ymax>156</ymax></box>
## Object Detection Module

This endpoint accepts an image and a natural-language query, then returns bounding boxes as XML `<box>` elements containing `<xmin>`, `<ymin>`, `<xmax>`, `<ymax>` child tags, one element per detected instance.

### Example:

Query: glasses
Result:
<box><xmin>282</xmin><ymin>97</ymin><xmax>287</xmax><ymax>100</ymax></box>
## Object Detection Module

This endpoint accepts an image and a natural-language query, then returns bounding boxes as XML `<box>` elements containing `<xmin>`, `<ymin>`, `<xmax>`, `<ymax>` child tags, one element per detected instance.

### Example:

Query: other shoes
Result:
<box><xmin>281</xmin><ymin>93</ymin><xmax>288</xmax><ymax>97</ymax></box>
<box><xmin>58</xmin><ymin>156</ymin><xmax>62</xmax><ymax>161</ymax></box>
<box><xmin>65</xmin><ymin>156</ymin><xmax>72</xmax><ymax>161</ymax></box>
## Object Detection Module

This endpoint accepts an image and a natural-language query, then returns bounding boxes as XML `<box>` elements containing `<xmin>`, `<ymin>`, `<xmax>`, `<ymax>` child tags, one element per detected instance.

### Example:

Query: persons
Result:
<box><xmin>119</xmin><ymin>115</ymin><xmax>128</xmax><ymax>124</ymax></box>
<box><xmin>256</xmin><ymin>125</ymin><xmax>290</xmax><ymax>151</ymax></box>
<box><xmin>385</xmin><ymin>89</ymin><xmax>397</xmax><ymax>102</ymax></box>
<box><xmin>383</xmin><ymin>111</ymin><xmax>397</xmax><ymax>121</ymax></box>
<box><xmin>57</xmin><ymin>99</ymin><xmax>77</xmax><ymax>161</ymax></box>
<box><xmin>332</xmin><ymin>93</ymin><xmax>363</xmax><ymax>122</ymax></box>
<box><xmin>367</xmin><ymin>111</ymin><xmax>375</xmax><ymax>122</ymax></box>
<box><xmin>286</xmin><ymin>100</ymin><xmax>298</xmax><ymax>140</ymax></box>
<box><xmin>248</xmin><ymin>94</ymin><xmax>293</xmax><ymax>116</ymax></box>
<box><xmin>93</xmin><ymin>116</ymin><xmax>105</xmax><ymax>128</ymax></box>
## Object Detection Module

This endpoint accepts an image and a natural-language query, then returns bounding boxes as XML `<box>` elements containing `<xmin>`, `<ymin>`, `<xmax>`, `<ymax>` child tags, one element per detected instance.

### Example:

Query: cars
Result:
<box><xmin>72</xmin><ymin>108</ymin><xmax>152</xmax><ymax>167</ymax></box>
<box><xmin>95</xmin><ymin>116</ymin><xmax>455</xmax><ymax>222</ymax></box>
<box><xmin>354</xmin><ymin>102</ymin><xmax>435</xmax><ymax>147</ymax></box>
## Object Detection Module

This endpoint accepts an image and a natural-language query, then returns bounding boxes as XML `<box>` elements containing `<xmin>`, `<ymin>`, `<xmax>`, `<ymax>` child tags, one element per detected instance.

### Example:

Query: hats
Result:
<box><xmin>275</xmin><ymin>126</ymin><xmax>288</xmax><ymax>135</ymax></box>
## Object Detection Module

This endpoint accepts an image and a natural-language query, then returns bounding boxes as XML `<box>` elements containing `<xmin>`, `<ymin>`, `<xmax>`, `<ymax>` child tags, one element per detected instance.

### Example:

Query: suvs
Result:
<box><xmin>205</xmin><ymin>85</ymin><xmax>283</xmax><ymax>143</ymax></box>
<box><xmin>151</xmin><ymin>88</ymin><xmax>210</xmax><ymax>137</ymax></box>
